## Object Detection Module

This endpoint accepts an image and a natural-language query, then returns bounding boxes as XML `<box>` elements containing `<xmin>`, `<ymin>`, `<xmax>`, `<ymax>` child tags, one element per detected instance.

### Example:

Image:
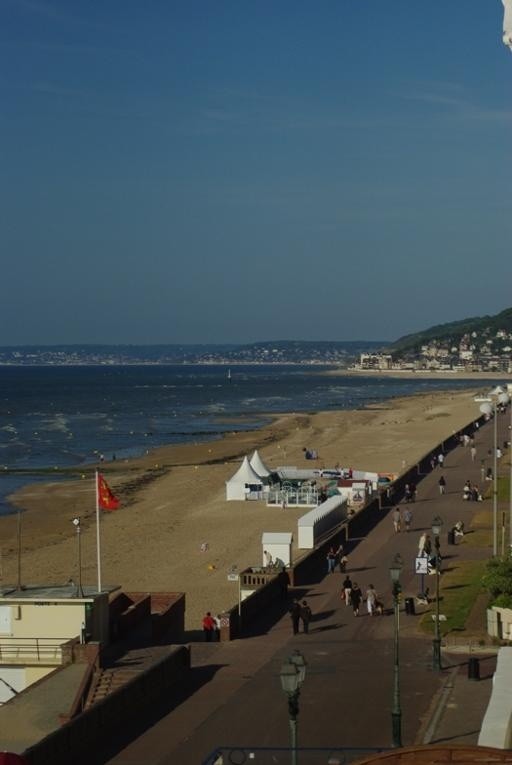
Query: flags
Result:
<box><xmin>99</xmin><ymin>474</ymin><xmax>118</xmax><ymax>510</ymax></box>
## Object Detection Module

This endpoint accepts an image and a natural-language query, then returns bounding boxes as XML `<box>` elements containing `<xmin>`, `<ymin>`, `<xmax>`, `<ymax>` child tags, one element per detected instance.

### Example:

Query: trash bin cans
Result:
<box><xmin>468</xmin><ymin>657</ymin><xmax>480</xmax><ymax>681</ymax></box>
<box><xmin>448</xmin><ymin>532</ymin><xmax>454</xmax><ymax>545</ymax></box>
<box><xmin>503</xmin><ymin>441</ymin><xmax>508</xmax><ymax>449</ymax></box>
<box><xmin>486</xmin><ymin>468</ymin><xmax>491</xmax><ymax>477</ymax></box>
<box><xmin>404</xmin><ymin>597</ymin><xmax>415</xmax><ymax>616</ymax></box>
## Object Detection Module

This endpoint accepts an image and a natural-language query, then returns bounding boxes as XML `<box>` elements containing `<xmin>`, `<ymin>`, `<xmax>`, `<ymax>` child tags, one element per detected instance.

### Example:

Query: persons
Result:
<box><xmin>288</xmin><ymin>428</ymin><xmax>512</xmax><ymax>633</ymax></box>
<box><xmin>99</xmin><ymin>452</ymin><xmax>105</xmax><ymax>464</ymax></box>
<box><xmin>213</xmin><ymin>614</ymin><xmax>221</xmax><ymax>641</ymax></box>
<box><xmin>201</xmin><ymin>611</ymin><xmax>217</xmax><ymax>642</ymax></box>
<box><xmin>475</xmin><ymin>406</ymin><xmax>511</xmax><ymax>431</ymax></box>
<box><xmin>263</xmin><ymin>550</ymin><xmax>274</xmax><ymax>566</ymax></box>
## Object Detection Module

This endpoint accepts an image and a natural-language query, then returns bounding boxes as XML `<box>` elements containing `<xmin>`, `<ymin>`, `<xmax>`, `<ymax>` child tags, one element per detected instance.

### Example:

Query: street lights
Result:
<box><xmin>72</xmin><ymin>516</ymin><xmax>84</xmax><ymax>598</ymax></box>
<box><xmin>387</xmin><ymin>550</ymin><xmax>407</xmax><ymax>750</ymax></box>
<box><xmin>277</xmin><ymin>648</ymin><xmax>308</xmax><ymax>765</ymax></box>
<box><xmin>429</xmin><ymin>517</ymin><xmax>446</xmax><ymax>672</ymax></box>
<box><xmin>479</xmin><ymin>392</ymin><xmax>510</xmax><ymax>555</ymax></box>
